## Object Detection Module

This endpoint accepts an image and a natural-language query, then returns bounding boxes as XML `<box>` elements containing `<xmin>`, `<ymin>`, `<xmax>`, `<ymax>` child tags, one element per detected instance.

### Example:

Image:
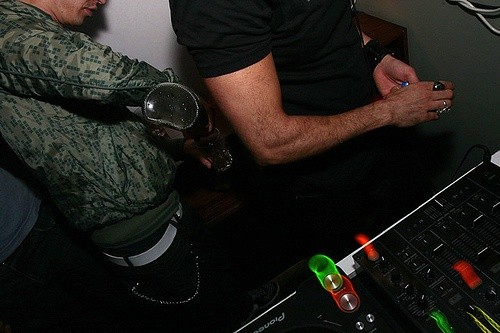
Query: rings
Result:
<box><xmin>433</xmin><ymin>80</ymin><xmax>445</xmax><ymax>91</ymax></box>
<box><xmin>437</xmin><ymin>99</ymin><xmax>451</xmax><ymax>114</ymax></box>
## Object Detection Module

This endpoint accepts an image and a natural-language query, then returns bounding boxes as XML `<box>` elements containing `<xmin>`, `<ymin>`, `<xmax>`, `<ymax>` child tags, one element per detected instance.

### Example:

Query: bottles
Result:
<box><xmin>143</xmin><ymin>83</ymin><xmax>215</xmax><ymax>139</ymax></box>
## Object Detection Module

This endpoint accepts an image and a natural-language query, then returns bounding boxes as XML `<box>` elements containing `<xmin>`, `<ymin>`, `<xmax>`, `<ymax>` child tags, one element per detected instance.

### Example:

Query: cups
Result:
<box><xmin>192</xmin><ymin>128</ymin><xmax>233</xmax><ymax>173</ymax></box>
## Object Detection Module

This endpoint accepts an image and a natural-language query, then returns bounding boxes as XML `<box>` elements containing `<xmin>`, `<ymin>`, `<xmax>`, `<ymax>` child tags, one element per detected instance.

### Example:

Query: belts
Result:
<box><xmin>102</xmin><ymin>202</ymin><xmax>184</xmax><ymax>267</ymax></box>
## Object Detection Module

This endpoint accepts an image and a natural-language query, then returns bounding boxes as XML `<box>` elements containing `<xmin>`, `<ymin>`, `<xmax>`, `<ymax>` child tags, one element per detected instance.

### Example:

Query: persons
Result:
<box><xmin>170</xmin><ymin>0</ymin><xmax>455</xmax><ymax>263</ymax></box>
<box><xmin>0</xmin><ymin>0</ymin><xmax>253</xmax><ymax>333</ymax></box>
<box><xmin>0</xmin><ymin>162</ymin><xmax>134</xmax><ymax>333</ymax></box>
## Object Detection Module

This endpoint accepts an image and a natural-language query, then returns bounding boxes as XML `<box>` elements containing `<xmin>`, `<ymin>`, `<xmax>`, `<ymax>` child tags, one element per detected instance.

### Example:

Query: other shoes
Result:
<box><xmin>235</xmin><ymin>280</ymin><xmax>279</xmax><ymax>327</ymax></box>
